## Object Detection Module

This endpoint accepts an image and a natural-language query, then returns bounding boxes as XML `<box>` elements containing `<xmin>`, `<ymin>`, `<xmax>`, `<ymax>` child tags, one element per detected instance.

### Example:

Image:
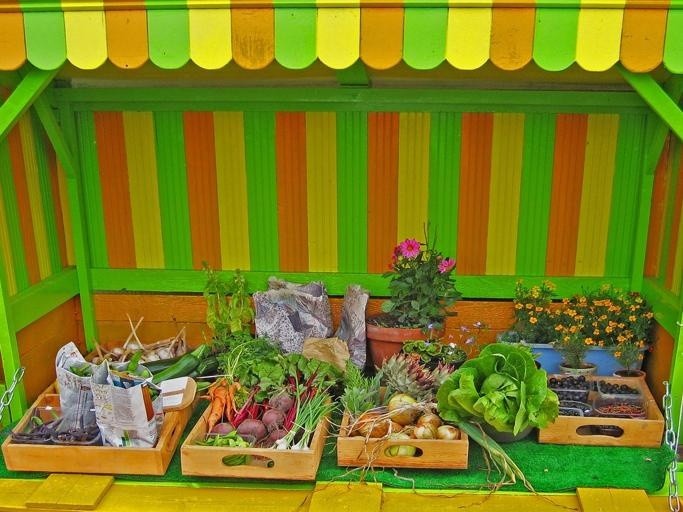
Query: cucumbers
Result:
<box><xmin>142</xmin><ymin>341</ymin><xmax>218</xmax><ymax>383</ymax></box>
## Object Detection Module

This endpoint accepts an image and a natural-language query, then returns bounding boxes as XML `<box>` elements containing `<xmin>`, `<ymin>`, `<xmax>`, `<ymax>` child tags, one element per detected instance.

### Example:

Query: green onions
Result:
<box><xmin>275</xmin><ymin>374</ymin><xmax>339</xmax><ymax>450</ymax></box>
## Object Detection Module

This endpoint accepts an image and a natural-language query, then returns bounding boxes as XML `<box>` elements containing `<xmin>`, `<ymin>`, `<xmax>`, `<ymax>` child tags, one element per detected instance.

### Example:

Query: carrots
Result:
<box><xmin>200</xmin><ymin>377</ymin><xmax>240</xmax><ymax>438</ymax></box>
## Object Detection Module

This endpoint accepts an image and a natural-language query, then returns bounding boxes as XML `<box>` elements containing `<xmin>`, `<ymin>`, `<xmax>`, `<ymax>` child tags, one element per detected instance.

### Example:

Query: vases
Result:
<box><xmin>561</xmin><ymin>363</ymin><xmax>597</xmax><ymax>374</ymax></box>
<box><xmin>495</xmin><ymin>333</ymin><xmax>652</xmax><ymax>375</ymax></box>
<box><xmin>365</xmin><ymin>314</ymin><xmax>445</xmax><ymax>386</ymax></box>
<box><xmin>417</xmin><ymin>346</ymin><xmax>468</xmax><ymax>374</ymax></box>
<box><xmin>613</xmin><ymin>368</ymin><xmax>647</xmax><ymax>376</ymax></box>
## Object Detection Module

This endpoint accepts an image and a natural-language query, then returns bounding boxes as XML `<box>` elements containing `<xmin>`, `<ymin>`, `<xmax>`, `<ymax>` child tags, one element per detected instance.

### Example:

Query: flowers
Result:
<box><xmin>379</xmin><ymin>223</ymin><xmax>463</xmax><ymax>327</ymax></box>
<box><xmin>513</xmin><ymin>278</ymin><xmax>656</xmax><ymax>370</ymax></box>
<box><xmin>401</xmin><ymin>320</ymin><xmax>484</xmax><ymax>363</ymax></box>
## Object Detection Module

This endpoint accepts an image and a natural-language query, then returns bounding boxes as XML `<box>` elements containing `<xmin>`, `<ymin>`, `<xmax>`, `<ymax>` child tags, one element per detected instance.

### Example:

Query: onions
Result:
<box><xmin>348</xmin><ymin>393</ymin><xmax>459</xmax><ymax>457</ymax></box>
